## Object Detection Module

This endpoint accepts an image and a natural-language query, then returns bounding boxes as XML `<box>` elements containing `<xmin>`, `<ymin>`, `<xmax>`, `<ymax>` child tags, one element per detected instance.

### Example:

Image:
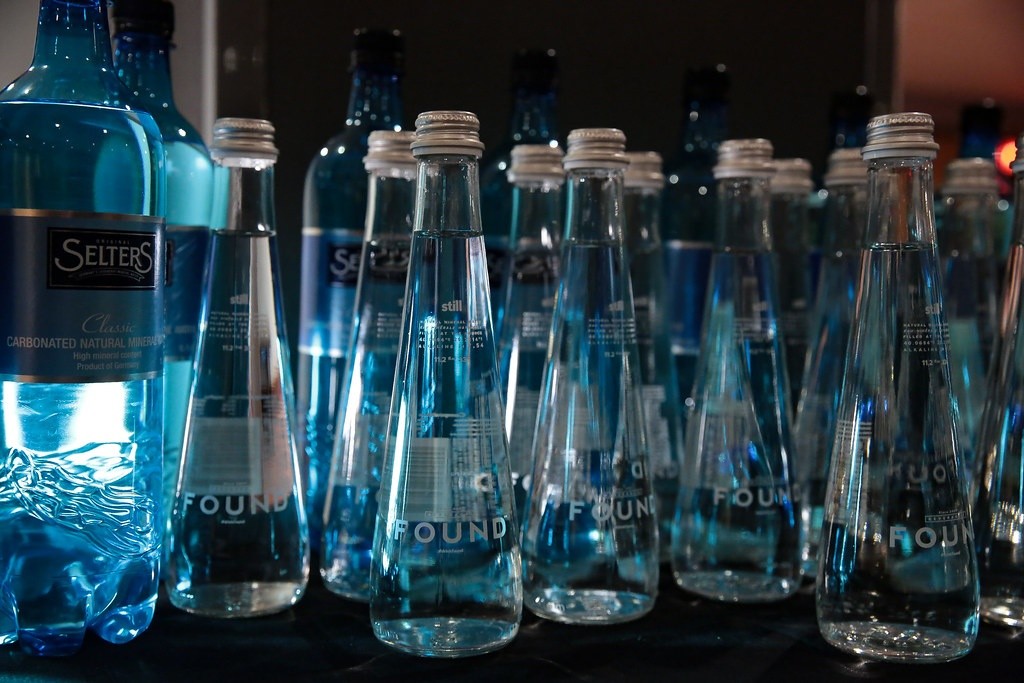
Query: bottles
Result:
<box><xmin>0</xmin><ymin>0</ymin><xmax>172</xmax><ymax>654</ymax></box>
<box><xmin>483</xmin><ymin>48</ymin><xmax>558</xmax><ymax>309</ymax></box>
<box><xmin>166</xmin><ymin>118</ymin><xmax>311</xmax><ymax>619</ymax></box>
<box><xmin>662</xmin><ymin>61</ymin><xmax>731</xmax><ymax>417</ymax></box>
<box><xmin>795</xmin><ymin>147</ymin><xmax>870</xmax><ymax>575</ymax></box>
<box><xmin>978</xmin><ymin>134</ymin><xmax>1024</xmax><ymax>626</ymax></box>
<box><xmin>106</xmin><ymin>0</ymin><xmax>213</xmax><ymax>566</ymax></box>
<box><xmin>496</xmin><ymin>145</ymin><xmax>564</xmax><ymax>522</ymax></box>
<box><xmin>320</xmin><ymin>131</ymin><xmax>486</xmax><ymax>597</ymax></box>
<box><xmin>370</xmin><ymin>111</ymin><xmax>525</xmax><ymax>659</ymax></box>
<box><xmin>815</xmin><ymin>90</ymin><xmax>873</xmax><ymax>305</ymax></box>
<box><xmin>957</xmin><ymin>104</ymin><xmax>1002</xmax><ymax>159</ymax></box>
<box><xmin>670</xmin><ymin>140</ymin><xmax>804</xmax><ymax>602</ymax></box>
<box><xmin>522</xmin><ymin>129</ymin><xmax>660</xmax><ymax>624</ymax></box>
<box><xmin>815</xmin><ymin>112</ymin><xmax>980</xmax><ymax>664</ymax></box>
<box><xmin>300</xmin><ymin>28</ymin><xmax>412</xmax><ymax>546</ymax></box>
<box><xmin>941</xmin><ymin>158</ymin><xmax>1000</xmax><ymax>484</ymax></box>
<box><xmin>624</xmin><ymin>152</ymin><xmax>679</xmax><ymax>565</ymax></box>
<box><xmin>771</xmin><ymin>160</ymin><xmax>812</xmax><ymax>417</ymax></box>
